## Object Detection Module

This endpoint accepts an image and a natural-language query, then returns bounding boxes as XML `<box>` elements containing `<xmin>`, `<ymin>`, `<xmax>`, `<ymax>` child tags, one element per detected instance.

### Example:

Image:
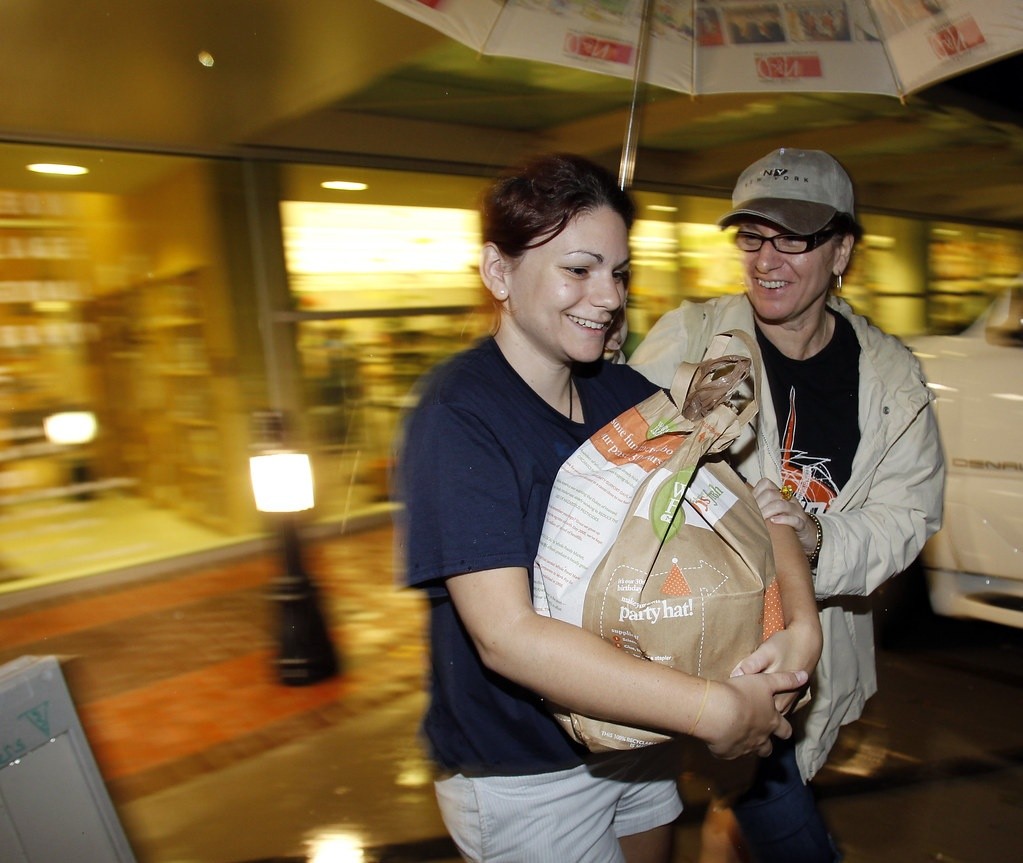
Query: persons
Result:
<box><xmin>604</xmin><ymin>148</ymin><xmax>947</xmax><ymax>863</ymax></box>
<box><xmin>400</xmin><ymin>157</ymin><xmax>823</xmax><ymax>863</ymax></box>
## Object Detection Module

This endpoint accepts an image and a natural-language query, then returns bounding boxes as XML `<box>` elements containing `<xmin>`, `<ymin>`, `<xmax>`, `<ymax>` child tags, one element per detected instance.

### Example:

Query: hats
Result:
<box><xmin>716</xmin><ymin>147</ymin><xmax>855</xmax><ymax>236</ymax></box>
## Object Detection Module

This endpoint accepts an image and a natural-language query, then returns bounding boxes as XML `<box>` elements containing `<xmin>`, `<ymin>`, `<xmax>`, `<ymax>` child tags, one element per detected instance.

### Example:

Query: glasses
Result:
<box><xmin>733</xmin><ymin>228</ymin><xmax>840</xmax><ymax>255</ymax></box>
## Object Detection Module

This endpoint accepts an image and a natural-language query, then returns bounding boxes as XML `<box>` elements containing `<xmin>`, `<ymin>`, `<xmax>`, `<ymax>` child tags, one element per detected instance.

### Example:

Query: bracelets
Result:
<box><xmin>805</xmin><ymin>512</ymin><xmax>822</xmax><ymax>562</ymax></box>
<box><xmin>688</xmin><ymin>678</ymin><xmax>710</xmax><ymax>735</ymax></box>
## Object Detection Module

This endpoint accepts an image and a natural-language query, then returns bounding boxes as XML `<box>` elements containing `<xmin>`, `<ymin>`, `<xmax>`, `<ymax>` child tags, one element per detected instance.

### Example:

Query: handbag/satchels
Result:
<box><xmin>530</xmin><ymin>331</ymin><xmax>812</xmax><ymax>754</ymax></box>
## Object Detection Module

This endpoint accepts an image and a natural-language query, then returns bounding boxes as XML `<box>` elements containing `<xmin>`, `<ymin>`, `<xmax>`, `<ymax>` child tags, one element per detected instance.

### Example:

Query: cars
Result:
<box><xmin>817</xmin><ymin>284</ymin><xmax>1023</xmax><ymax>650</ymax></box>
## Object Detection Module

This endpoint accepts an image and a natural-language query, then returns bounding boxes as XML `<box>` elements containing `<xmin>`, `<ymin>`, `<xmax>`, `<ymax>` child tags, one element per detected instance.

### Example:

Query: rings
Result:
<box><xmin>780</xmin><ymin>485</ymin><xmax>793</xmax><ymax>501</ymax></box>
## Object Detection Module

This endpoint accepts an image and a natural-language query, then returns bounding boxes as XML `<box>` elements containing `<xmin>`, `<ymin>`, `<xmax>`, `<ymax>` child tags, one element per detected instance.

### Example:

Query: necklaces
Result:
<box><xmin>568</xmin><ymin>377</ymin><xmax>574</xmax><ymax>420</ymax></box>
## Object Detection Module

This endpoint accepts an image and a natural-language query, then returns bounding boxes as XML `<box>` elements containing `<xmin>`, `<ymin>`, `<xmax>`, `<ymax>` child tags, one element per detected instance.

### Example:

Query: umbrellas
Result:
<box><xmin>374</xmin><ymin>0</ymin><xmax>1023</xmax><ymax>191</ymax></box>
